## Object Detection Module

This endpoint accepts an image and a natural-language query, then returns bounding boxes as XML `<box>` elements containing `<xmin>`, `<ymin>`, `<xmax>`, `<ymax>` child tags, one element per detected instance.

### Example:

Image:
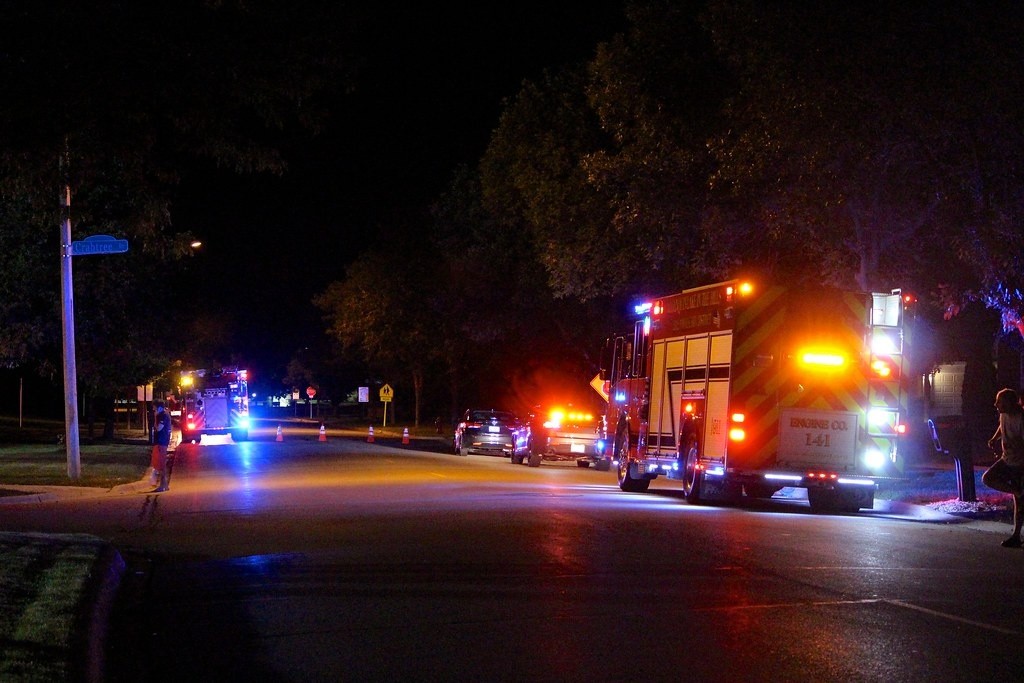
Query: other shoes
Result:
<box><xmin>154</xmin><ymin>487</ymin><xmax>165</xmax><ymax>492</ymax></box>
<box><xmin>1001</xmin><ymin>538</ymin><xmax>1021</xmax><ymax>546</ymax></box>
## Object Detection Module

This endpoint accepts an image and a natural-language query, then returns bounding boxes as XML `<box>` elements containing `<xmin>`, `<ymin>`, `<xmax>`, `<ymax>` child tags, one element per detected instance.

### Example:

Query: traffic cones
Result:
<box><xmin>317</xmin><ymin>423</ymin><xmax>327</xmax><ymax>442</ymax></box>
<box><xmin>400</xmin><ymin>426</ymin><xmax>410</xmax><ymax>446</ymax></box>
<box><xmin>275</xmin><ymin>424</ymin><xmax>284</xmax><ymax>442</ymax></box>
<box><xmin>365</xmin><ymin>425</ymin><xmax>376</xmax><ymax>443</ymax></box>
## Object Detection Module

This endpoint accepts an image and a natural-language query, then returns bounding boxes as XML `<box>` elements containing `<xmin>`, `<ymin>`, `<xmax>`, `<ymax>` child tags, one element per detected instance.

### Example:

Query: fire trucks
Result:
<box><xmin>599</xmin><ymin>270</ymin><xmax>917</xmax><ymax>517</ymax></box>
<box><xmin>178</xmin><ymin>367</ymin><xmax>250</xmax><ymax>443</ymax></box>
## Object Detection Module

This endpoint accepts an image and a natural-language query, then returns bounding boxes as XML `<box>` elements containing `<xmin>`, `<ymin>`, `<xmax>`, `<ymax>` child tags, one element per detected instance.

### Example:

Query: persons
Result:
<box><xmin>152</xmin><ymin>398</ymin><xmax>171</xmax><ymax>492</ymax></box>
<box><xmin>982</xmin><ymin>389</ymin><xmax>1024</xmax><ymax>547</ymax></box>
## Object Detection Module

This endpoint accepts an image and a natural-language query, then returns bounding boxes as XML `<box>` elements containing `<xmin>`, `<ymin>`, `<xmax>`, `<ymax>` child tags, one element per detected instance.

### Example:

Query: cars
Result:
<box><xmin>511</xmin><ymin>403</ymin><xmax>607</xmax><ymax>469</ymax></box>
<box><xmin>454</xmin><ymin>408</ymin><xmax>521</xmax><ymax>457</ymax></box>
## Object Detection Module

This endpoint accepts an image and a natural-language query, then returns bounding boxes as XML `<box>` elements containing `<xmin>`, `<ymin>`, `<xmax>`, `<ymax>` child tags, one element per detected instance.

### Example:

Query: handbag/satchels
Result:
<box><xmin>152</xmin><ymin>445</ymin><xmax>160</xmax><ymax>470</ymax></box>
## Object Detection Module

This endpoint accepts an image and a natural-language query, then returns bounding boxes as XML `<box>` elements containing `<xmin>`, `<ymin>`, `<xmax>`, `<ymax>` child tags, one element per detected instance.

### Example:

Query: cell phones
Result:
<box><xmin>157</xmin><ymin>406</ymin><xmax>163</xmax><ymax>413</ymax></box>
